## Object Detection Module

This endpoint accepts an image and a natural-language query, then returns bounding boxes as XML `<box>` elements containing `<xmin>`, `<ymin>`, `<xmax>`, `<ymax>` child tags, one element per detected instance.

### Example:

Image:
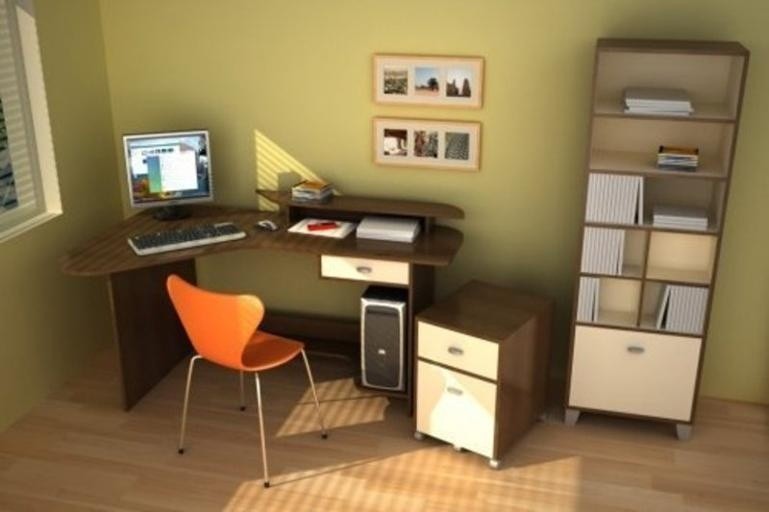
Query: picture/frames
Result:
<box><xmin>373</xmin><ymin>117</ymin><xmax>482</xmax><ymax>173</ymax></box>
<box><xmin>372</xmin><ymin>53</ymin><xmax>485</xmax><ymax>110</ymax></box>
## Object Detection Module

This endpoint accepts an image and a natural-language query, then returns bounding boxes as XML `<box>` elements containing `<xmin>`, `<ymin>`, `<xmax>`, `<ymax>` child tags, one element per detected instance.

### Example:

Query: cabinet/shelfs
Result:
<box><xmin>413</xmin><ymin>278</ymin><xmax>555</xmax><ymax>470</ymax></box>
<box><xmin>564</xmin><ymin>39</ymin><xmax>751</xmax><ymax>442</ymax></box>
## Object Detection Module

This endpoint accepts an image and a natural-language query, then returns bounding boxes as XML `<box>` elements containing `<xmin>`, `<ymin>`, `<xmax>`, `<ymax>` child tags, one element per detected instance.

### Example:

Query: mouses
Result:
<box><xmin>255</xmin><ymin>219</ymin><xmax>279</xmax><ymax>231</ymax></box>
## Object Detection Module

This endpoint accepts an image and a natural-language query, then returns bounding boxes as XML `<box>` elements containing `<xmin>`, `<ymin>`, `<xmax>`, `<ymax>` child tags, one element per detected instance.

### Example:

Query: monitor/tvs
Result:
<box><xmin>123</xmin><ymin>130</ymin><xmax>215</xmax><ymax>221</ymax></box>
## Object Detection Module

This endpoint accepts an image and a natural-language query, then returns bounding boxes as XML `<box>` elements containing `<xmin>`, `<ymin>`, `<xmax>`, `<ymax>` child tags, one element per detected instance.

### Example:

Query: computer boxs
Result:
<box><xmin>359</xmin><ymin>285</ymin><xmax>409</xmax><ymax>391</ymax></box>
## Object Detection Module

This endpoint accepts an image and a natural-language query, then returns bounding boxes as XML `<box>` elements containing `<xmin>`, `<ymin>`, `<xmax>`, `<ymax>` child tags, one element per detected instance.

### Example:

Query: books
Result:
<box><xmin>291</xmin><ymin>180</ymin><xmax>332</xmax><ymax>202</ymax></box>
<box><xmin>622</xmin><ymin>87</ymin><xmax>696</xmax><ymax>119</ymax></box>
<box><xmin>288</xmin><ymin>214</ymin><xmax>421</xmax><ymax>243</ymax></box>
<box><xmin>655</xmin><ymin>144</ymin><xmax>701</xmax><ymax>171</ymax></box>
<box><xmin>573</xmin><ymin>172</ymin><xmax>712</xmax><ymax>337</ymax></box>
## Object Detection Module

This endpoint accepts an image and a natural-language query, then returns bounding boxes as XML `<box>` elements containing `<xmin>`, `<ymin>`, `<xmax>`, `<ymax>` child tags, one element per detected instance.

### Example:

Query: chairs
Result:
<box><xmin>166</xmin><ymin>275</ymin><xmax>327</xmax><ymax>487</ymax></box>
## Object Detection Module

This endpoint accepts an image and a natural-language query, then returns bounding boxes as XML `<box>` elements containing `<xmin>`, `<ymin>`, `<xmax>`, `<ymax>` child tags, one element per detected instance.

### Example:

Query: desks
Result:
<box><xmin>56</xmin><ymin>190</ymin><xmax>464</xmax><ymax>416</ymax></box>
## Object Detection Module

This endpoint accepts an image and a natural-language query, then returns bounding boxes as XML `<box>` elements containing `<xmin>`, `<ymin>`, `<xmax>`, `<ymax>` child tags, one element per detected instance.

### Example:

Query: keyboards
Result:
<box><xmin>127</xmin><ymin>221</ymin><xmax>247</xmax><ymax>258</ymax></box>
